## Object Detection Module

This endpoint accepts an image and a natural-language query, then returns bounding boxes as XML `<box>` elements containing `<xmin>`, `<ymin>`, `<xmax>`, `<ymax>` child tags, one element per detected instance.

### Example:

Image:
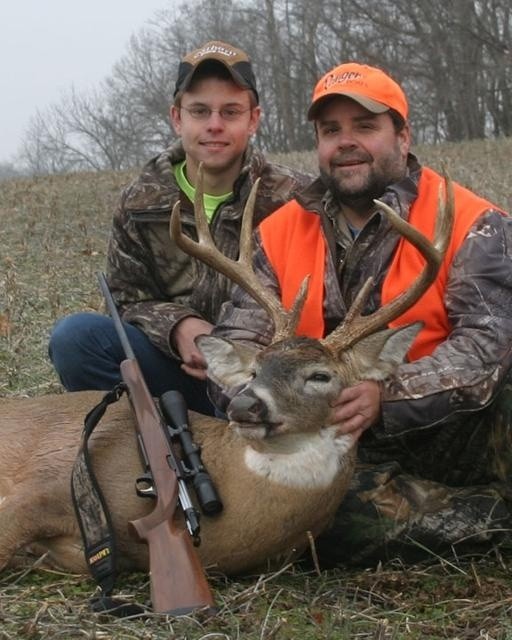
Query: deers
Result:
<box><xmin>0</xmin><ymin>157</ymin><xmax>455</xmax><ymax>584</ymax></box>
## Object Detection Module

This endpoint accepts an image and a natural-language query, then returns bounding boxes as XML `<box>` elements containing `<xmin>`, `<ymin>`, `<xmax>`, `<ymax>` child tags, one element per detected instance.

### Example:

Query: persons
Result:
<box><xmin>217</xmin><ymin>61</ymin><xmax>511</xmax><ymax>571</ymax></box>
<box><xmin>46</xmin><ymin>40</ymin><xmax>319</xmax><ymax>418</ymax></box>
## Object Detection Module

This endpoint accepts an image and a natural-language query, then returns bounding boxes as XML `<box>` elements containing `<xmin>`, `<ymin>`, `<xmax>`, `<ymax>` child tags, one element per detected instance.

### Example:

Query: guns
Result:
<box><xmin>94</xmin><ymin>270</ymin><xmax>222</xmax><ymax>624</ymax></box>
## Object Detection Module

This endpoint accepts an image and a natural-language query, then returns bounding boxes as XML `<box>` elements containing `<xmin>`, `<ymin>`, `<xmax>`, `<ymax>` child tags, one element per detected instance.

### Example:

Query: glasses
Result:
<box><xmin>182</xmin><ymin>105</ymin><xmax>250</xmax><ymax>119</ymax></box>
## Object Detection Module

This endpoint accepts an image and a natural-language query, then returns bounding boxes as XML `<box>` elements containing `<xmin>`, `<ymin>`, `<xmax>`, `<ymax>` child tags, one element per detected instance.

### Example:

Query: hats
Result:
<box><xmin>308</xmin><ymin>63</ymin><xmax>408</xmax><ymax>124</ymax></box>
<box><xmin>174</xmin><ymin>40</ymin><xmax>260</xmax><ymax>104</ymax></box>
